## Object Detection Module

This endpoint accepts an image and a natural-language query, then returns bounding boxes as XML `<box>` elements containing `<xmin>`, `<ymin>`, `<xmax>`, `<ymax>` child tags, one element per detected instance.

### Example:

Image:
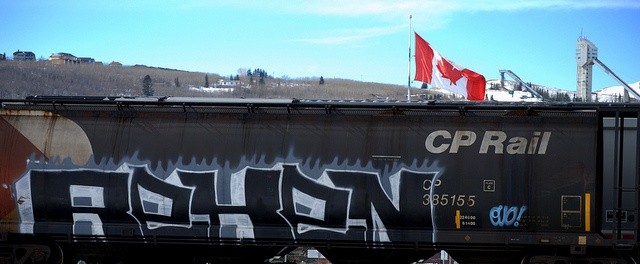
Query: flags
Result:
<box><xmin>413</xmin><ymin>31</ymin><xmax>486</xmax><ymax>101</ymax></box>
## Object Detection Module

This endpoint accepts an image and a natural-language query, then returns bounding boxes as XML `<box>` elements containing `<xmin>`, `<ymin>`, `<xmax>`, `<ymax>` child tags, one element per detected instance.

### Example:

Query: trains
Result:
<box><xmin>0</xmin><ymin>94</ymin><xmax>639</xmax><ymax>262</ymax></box>
